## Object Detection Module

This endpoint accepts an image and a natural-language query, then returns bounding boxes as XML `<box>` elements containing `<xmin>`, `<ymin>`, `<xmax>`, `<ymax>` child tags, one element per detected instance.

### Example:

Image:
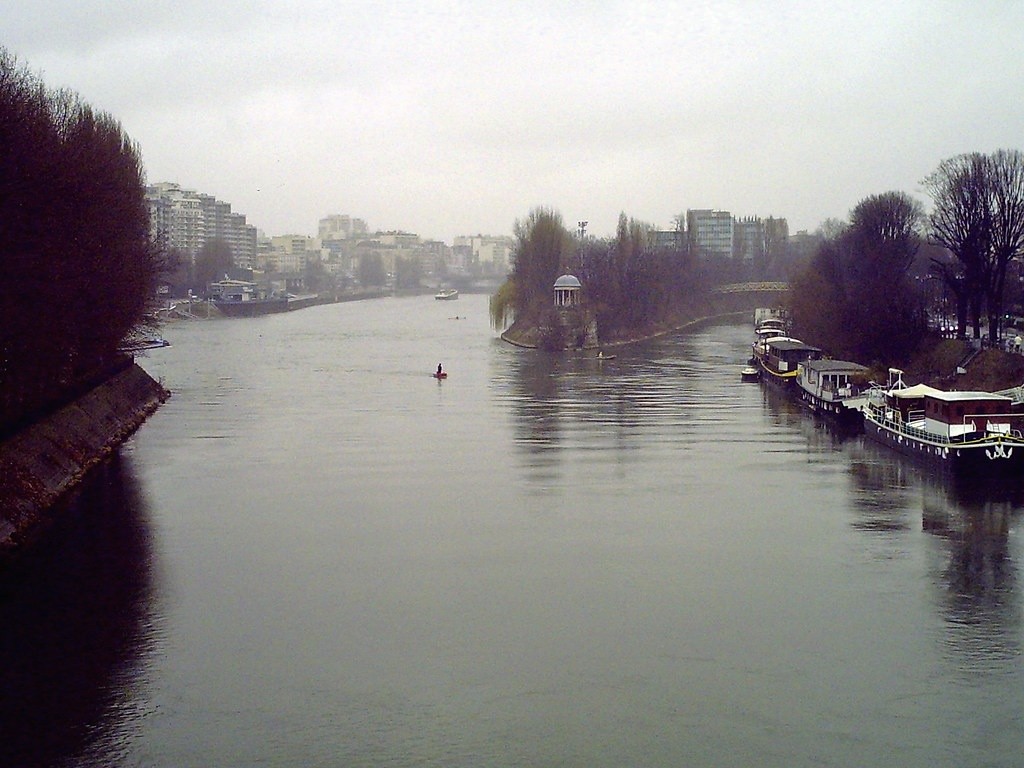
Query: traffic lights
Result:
<box><xmin>1004</xmin><ymin>311</ymin><xmax>1010</xmax><ymax>319</ymax></box>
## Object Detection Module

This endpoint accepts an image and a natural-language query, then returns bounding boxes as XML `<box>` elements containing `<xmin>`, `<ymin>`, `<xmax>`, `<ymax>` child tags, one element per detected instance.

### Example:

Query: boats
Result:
<box><xmin>741</xmin><ymin>365</ymin><xmax>758</xmax><ymax>383</ymax></box>
<box><xmin>435</xmin><ymin>290</ymin><xmax>458</xmax><ymax>300</ymax></box>
<box><xmin>757</xmin><ymin>337</ymin><xmax>823</xmax><ymax>392</ymax></box>
<box><xmin>116</xmin><ymin>338</ymin><xmax>170</xmax><ymax>352</ymax></box>
<box><xmin>795</xmin><ymin>356</ymin><xmax>880</xmax><ymax>428</ymax></box>
<box><xmin>595</xmin><ymin>354</ymin><xmax>616</xmax><ymax>360</ymax></box>
<box><xmin>433</xmin><ymin>373</ymin><xmax>447</xmax><ymax>378</ymax></box>
<box><xmin>748</xmin><ymin>315</ymin><xmax>805</xmax><ymax>368</ymax></box>
<box><xmin>861</xmin><ymin>368</ymin><xmax>1024</xmax><ymax>474</ymax></box>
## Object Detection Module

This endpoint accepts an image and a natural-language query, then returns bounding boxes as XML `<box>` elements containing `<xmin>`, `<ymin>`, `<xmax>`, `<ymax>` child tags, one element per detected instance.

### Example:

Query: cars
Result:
<box><xmin>966</xmin><ymin>316</ymin><xmax>983</xmax><ymax>327</ymax></box>
<box><xmin>928</xmin><ymin>314</ymin><xmax>958</xmax><ymax>333</ymax></box>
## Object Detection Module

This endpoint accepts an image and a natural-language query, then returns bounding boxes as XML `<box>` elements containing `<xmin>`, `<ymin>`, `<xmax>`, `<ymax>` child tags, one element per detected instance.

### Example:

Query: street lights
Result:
<box><xmin>926</xmin><ymin>274</ymin><xmax>964</xmax><ymax>340</ymax></box>
